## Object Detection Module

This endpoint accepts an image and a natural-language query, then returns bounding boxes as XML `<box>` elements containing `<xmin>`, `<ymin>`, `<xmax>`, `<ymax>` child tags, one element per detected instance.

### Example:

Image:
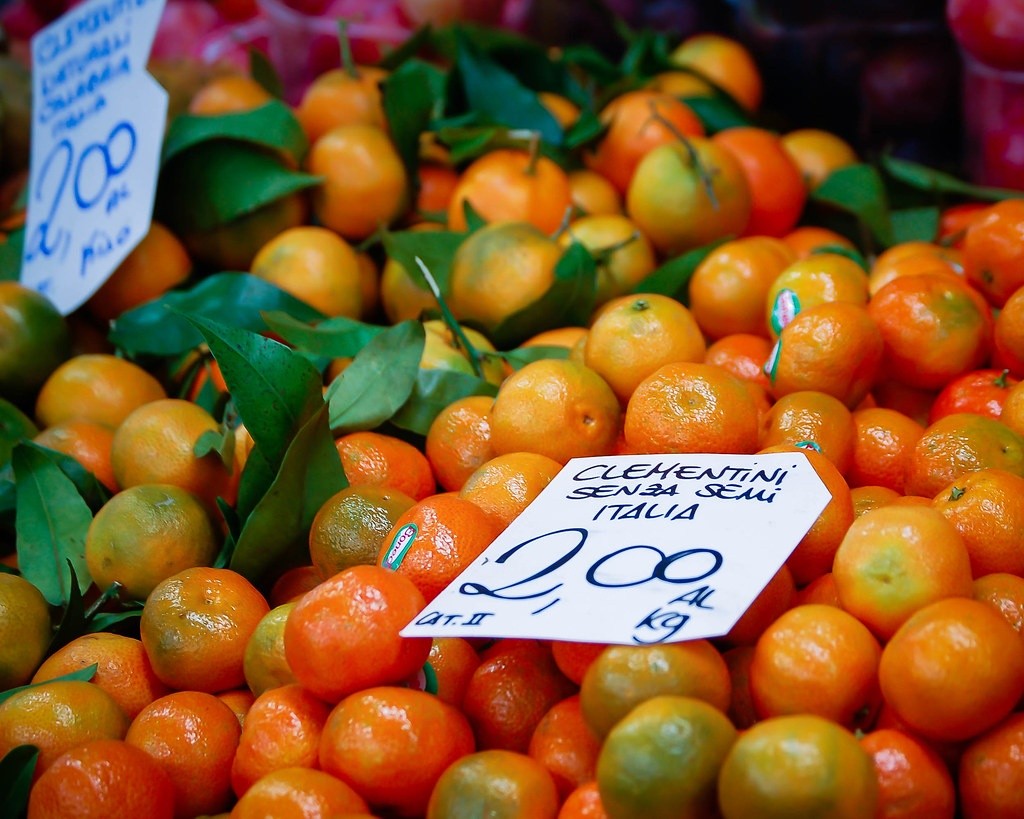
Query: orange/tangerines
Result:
<box><xmin>0</xmin><ymin>37</ymin><xmax>1024</xmax><ymax>819</ymax></box>
